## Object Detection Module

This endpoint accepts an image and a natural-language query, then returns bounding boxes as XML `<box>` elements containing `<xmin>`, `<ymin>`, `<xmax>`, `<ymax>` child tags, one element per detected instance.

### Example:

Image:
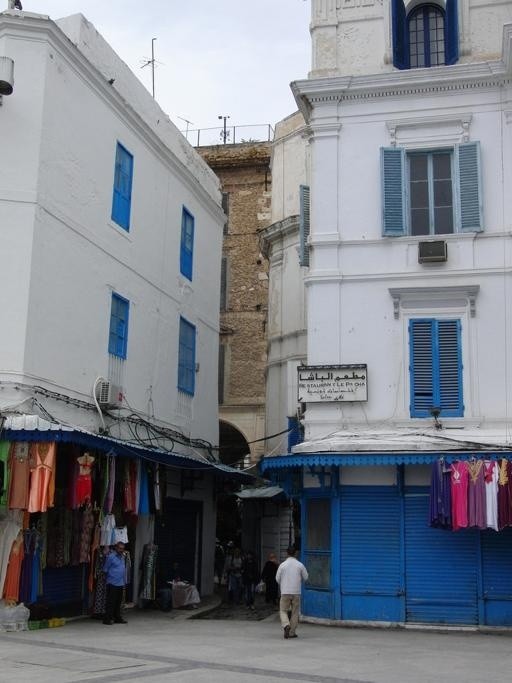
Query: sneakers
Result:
<box><xmin>284</xmin><ymin>626</ymin><xmax>297</xmax><ymax>638</ymax></box>
<box><xmin>114</xmin><ymin>619</ymin><xmax>127</xmax><ymax>623</ymax></box>
<box><xmin>103</xmin><ymin>620</ymin><xmax>112</xmax><ymax>624</ymax></box>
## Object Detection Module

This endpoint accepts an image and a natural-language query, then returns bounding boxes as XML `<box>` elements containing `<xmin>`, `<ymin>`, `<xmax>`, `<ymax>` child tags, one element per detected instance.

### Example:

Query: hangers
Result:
<box><xmin>469</xmin><ymin>456</ymin><xmax>491</xmax><ymax>464</ymax></box>
<box><xmin>106</xmin><ymin>448</ymin><xmax>117</xmax><ymax>456</ymax></box>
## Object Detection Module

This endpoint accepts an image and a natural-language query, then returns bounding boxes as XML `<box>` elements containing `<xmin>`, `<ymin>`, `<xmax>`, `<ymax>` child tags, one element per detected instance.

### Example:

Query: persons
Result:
<box><xmin>94</xmin><ymin>546</ymin><xmax>113</xmax><ymax>614</ymax></box>
<box><xmin>77</xmin><ymin>452</ymin><xmax>95</xmax><ymax>476</ymax></box>
<box><xmin>102</xmin><ymin>541</ymin><xmax>128</xmax><ymax>624</ymax></box>
<box><xmin>274</xmin><ymin>545</ymin><xmax>309</xmax><ymax>639</ymax></box>
<box><xmin>214</xmin><ymin>546</ymin><xmax>280</xmax><ymax>609</ymax></box>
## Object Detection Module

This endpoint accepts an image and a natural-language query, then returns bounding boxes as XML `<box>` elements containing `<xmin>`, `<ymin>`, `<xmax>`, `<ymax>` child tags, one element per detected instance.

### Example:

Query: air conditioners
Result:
<box><xmin>100</xmin><ymin>381</ymin><xmax>123</xmax><ymax>406</ymax></box>
<box><xmin>417</xmin><ymin>242</ymin><xmax>448</xmax><ymax>263</ymax></box>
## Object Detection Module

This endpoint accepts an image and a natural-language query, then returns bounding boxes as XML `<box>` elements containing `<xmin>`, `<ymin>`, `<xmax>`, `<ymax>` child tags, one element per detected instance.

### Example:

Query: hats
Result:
<box><xmin>216</xmin><ymin>538</ymin><xmax>220</xmax><ymax>542</ymax></box>
<box><xmin>228</xmin><ymin>541</ymin><xmax>233</xmax><ymax>546</ymax></box>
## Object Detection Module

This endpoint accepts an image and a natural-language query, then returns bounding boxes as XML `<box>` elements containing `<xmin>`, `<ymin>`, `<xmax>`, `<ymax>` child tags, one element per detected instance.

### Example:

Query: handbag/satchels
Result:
<box><xmin>259</xmin><ymin>582</ymin><xmax>266</xmax><ymax>593</ymax></box>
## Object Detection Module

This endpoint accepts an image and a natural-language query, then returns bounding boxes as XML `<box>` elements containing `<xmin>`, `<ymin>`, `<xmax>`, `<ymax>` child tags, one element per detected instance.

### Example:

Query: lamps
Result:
<box><xmin>431</xmin><ymin>408</ymin><xmax>442</xmax><ymax>429</ymax></box>
<box><xmin>0</xmin><ymin>56</ymin><xmax>14</xmax><ymax>104</ymax></box>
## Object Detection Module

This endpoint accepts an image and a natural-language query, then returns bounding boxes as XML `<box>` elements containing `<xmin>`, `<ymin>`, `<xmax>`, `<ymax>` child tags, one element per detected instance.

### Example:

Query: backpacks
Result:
<box><xmin>216</xmin><ymin>546</ymin><xmax>223</xmax><ymax>560</ymax></box>
